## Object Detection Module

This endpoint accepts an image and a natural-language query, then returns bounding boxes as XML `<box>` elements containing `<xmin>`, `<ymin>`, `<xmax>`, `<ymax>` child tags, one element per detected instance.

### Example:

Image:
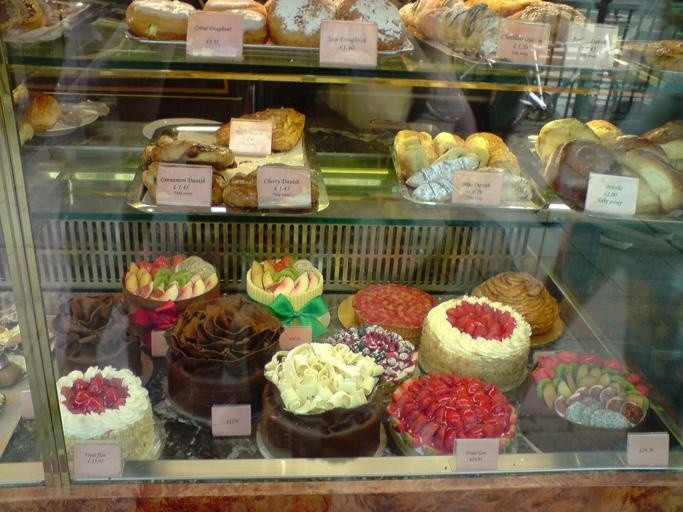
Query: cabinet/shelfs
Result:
<box><xmin>0</xmin><ymin>0</ymin><xmax>683</xmax><ymax>512</ymax></box>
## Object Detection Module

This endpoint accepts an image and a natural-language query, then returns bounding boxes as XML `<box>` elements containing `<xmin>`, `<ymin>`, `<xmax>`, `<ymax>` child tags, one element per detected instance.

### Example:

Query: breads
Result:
<box><xmin>11</xmin><ymin>83</ymin><xmax>27</xmax><ymax>103</ymax></box>
<box><xmin>222</xmin><ymin>164</ymin><xmax>319</xmax><ymax>213</ymax></box>
<box><xmin>399</xmin><ymin>0</ymin><xmax>621</xmax><ymax>66</ymax></box>
<box><xmin>465</xmin><ymin>133</ymin><xmax>505</xmax><ymax>152</ymax></box>
<box><xmin>202</xmin><ymin>0</ymin><xmax>262</xmax><ymax>11</ymax></box>
<box><xmin>394</xmin><ymin>130</ymin><xmax>435</xmax><ymax>178</ymax></box>
<box><xmin>263</xmin><ymin>0</ymin><xmax>336</xmax><ymax>48</ymax></box>
<box><xmin>153</xmin><ymin>142</ymin><xmax>234</xmax><ymax>170</ymax></box>
<box><xmin>434</xmin><ymin>132</ymin><xmax>465</xmax><ymax>158</ymax></box>
<box><xmin>544</xmin><ymin>141</ymin><xmax>621</xmax><ymax>208</ymax></box>
<box><xmin>405</xmin><ymin>154</ymin><xmax>479</xmax><ymax>188</ymax></box>
<box><xmin>471</xmin><ymin>271</ymin><xmax>560</xmax><ymax>336</ymax></box>
<box><xmin>223</xmin><ymin>10</ymin><xmax>267</xmax><ymax>43</ymax></box>
<box><xmin>474</xmin><ymin>168</ymin><xmax>531</xmax><ymax>205</ymax></box>
<box><xmin>641</xmin><ymin>123</ymin><xmax>683</xmax><ymax>164</ymax></box>
<box><xmin>644</xmin><ymin>41</ymin><xmax>683</xmax><ymax>71</ymax></box>
<box><xmin>126</xmin><ymin>0</ymin><xmax>196</xmax><ymax>42</ymax></box>
<box><xmin>584</xmin><ymin>119</ymin><xmax>622</xmax><ymax>142</ymax></box>
<box><xmin>616</xmin><ymin>166</ymin><xmax>660</xmax><ymax>215</ymax></box>
<box><xmin>414</xmin><ymin>178</ymin><xmax>452</xmax><ymax>202</ymax></box>
<box><xmin>336</xmin><ymin>0</ymin><xmax>405</xmax><ymax>52</ymax></box>
<box><xmin>617</xmin><ymin>150</ymin><xmax>683</xmax><ymax>212</ymax></box>
<box><xmin>216</xmin><ymin>108</ymin><xmax>304</xmax><ymax>152</ymax></box>
<box><xmin>536</xmin><ymin>117</ymin><xmax>598</xmax><ymax>157</ymax></box>
<box><xmin>603</xmin><ymin>136</ymin><xmax>667</xmax><ymax>166</ymax></box>
<box><xmin>140</xmin><ymin>161</ymin><xmax>223</xmax><ymax>207</ymax></box>
<box><xmin>488</xmin><ymin>150</ymin><xmax>520</xmax><ymax>177</ymax></box>
<box><xmin>16</xmin><ymin>123</ymin><xmax>30</xmax><ymax>147</ymax></box>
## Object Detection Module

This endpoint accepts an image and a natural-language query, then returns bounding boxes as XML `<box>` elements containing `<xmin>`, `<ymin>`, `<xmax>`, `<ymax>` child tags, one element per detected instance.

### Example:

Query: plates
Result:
<box><xmin>142</xmin><ymin>118</ymin><xmax>223</xmax><ymax>142</ymax></box>
<box><xmin>1</xmin><ymin>0</ymin><xmax>101</xmax><ymax>45</ymax></box>
<box><xmin>125</xmin><ymin>122</ymin><xmax>330</xmax><ymax>217</ymax></box>
<box><xmin>125</xmin><ymin>27</ymin><xmax>415</xmax><ymax>58</ymax></box>
<box><xmin>388</xmin><ymin>140</ymin><xmax>548</xmax><ymax>213</ymax></box>
<box><xmin>412</xmin><ymin>30</ymin><xmax>634</xmax><ymax>72</ymax></box>
<box><xmin>524</xmin><ymin>132</ymin><xmax>683</xmax><ymax>224</ymax></box>
<box><xmin>32</xmin><ymin>101</ymin><xmax>99</xmax><ymax>136</ymax></box>
<box><xmin>613</xmin><ymin>58</ymin><xmax>683</xmax><ymax>96</ymax></box>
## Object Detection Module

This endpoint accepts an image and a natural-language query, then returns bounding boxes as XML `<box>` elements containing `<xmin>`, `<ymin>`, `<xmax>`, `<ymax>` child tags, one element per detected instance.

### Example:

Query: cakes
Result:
<box><xmin>51</xmin><ymin>297</ymin><xmax>142</xmax><ymax>380</ymax></box>
<box><xmin>260</xmin><ymin>343</ymin><xmax>383</xmax><ymax>458</ymax></box>
<box><xmin>0</xmin><ymin>1</ymin><xmax>47</xmax><ymax>37</ymax></box>
<box><xmin>166</xmin><ymin>298</ymin><xmax>284</xmax><ymax>421</ymax></box>
<box><xmin>420</xmin><ymin>295</ymin><xmax>532</xmax><ymax>392</ymax></box>
<box><xmin>354</xmin><ymin>284</ymin><xmax>436</xmax><ymax>340</ymax></box>
<box><xmin>518</xmin><ymin>352</ymin><xmax>650</xmax><ymax>452</ymax></box>
<box><xmin>323</xmin><ymin>324</ymin><xmax>419</xmax><ymax>409</ymax></box>
<box><xmin>121</xmin><ymin>255</ymin><xmax>218</xmax><ymax>349</ymax></box>
<box><xmin>386</xmin><ymin>374</ymin><xmax>518</xmax><ymax>456</ymax></box>
<box><xmin>246</xmin><ymin>258</ymin><xmax>330</xmax><ymax>342</ymax></box>
<box><xmin>56</xmin><ymin>367</ymin><xmax>161</xmax><ymax>464</ymax></box>
